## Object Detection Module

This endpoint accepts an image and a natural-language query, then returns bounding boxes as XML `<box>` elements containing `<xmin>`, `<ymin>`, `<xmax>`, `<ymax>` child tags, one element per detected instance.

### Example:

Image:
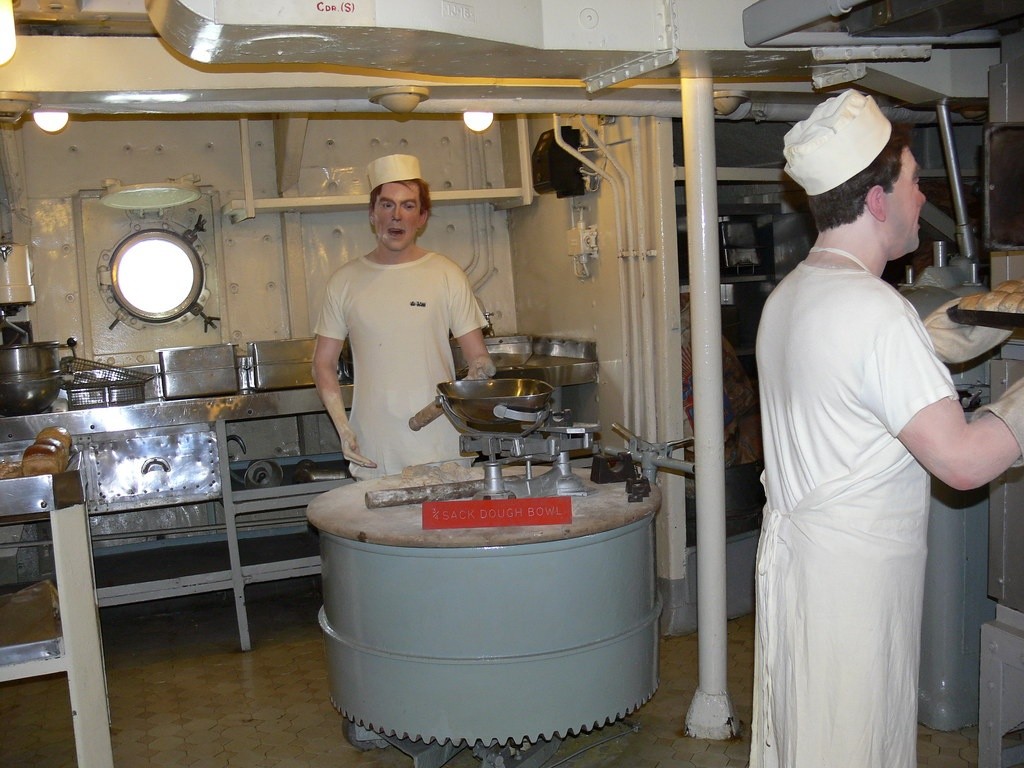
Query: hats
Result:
<box><xmin>366</xmin><ymin>155</ymin><xmax>420</xmax><ymax>193</ymax></box>
<box><xmin>782</xmin><ymin>89</ymin><xmax>892</xmax><ymax>197</ymax></box>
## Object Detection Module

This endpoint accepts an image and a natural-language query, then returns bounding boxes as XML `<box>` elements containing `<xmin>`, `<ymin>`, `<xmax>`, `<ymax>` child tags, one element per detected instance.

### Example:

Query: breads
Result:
<box><xmin>957</xmin><ymin>276</ymin><xmax>1024</xmax><ymax>313</ymax></box>
<box><xmin>0</xmin><ymin>425</ymin><xmax>72</xmax><ymax>479</ymax></box>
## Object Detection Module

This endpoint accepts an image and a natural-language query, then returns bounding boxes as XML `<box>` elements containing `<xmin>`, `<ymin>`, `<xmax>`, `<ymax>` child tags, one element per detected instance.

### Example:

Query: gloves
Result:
<box><xmin>920</xmin><ymin>279</ymin><xmax>1024</xmax><ymax>365</ymax></box>
<box><xmin>970</xmin><ymin>376</ymin><xmax>1023</xmax><ymax>468</ymax></box>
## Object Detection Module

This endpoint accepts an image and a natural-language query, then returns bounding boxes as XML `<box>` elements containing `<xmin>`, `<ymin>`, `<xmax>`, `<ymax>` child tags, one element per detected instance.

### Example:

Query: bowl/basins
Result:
<box><xmin>435</xmin><ymin>378</ymin><xmax>558</xmax><ymax>425</ymax></box>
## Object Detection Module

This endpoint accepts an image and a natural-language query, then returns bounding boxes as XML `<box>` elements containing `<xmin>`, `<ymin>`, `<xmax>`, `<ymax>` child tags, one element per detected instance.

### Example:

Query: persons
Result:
<box><xmin>311</xmin><ymin>153</ymin><xmax>494</xmax><ymax>484</ymax></box>
<box><xmin>748</xmin><ymin>89</ymin><xmax>1024</xmax><ymax>768</ymax></box>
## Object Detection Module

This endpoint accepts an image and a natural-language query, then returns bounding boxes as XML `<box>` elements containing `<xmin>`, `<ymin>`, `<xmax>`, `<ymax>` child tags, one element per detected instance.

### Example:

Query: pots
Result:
<box><xmin>0</xmin><ymin>336</ymin><xmax>77</xmax><ymax>416</ymax></box>
